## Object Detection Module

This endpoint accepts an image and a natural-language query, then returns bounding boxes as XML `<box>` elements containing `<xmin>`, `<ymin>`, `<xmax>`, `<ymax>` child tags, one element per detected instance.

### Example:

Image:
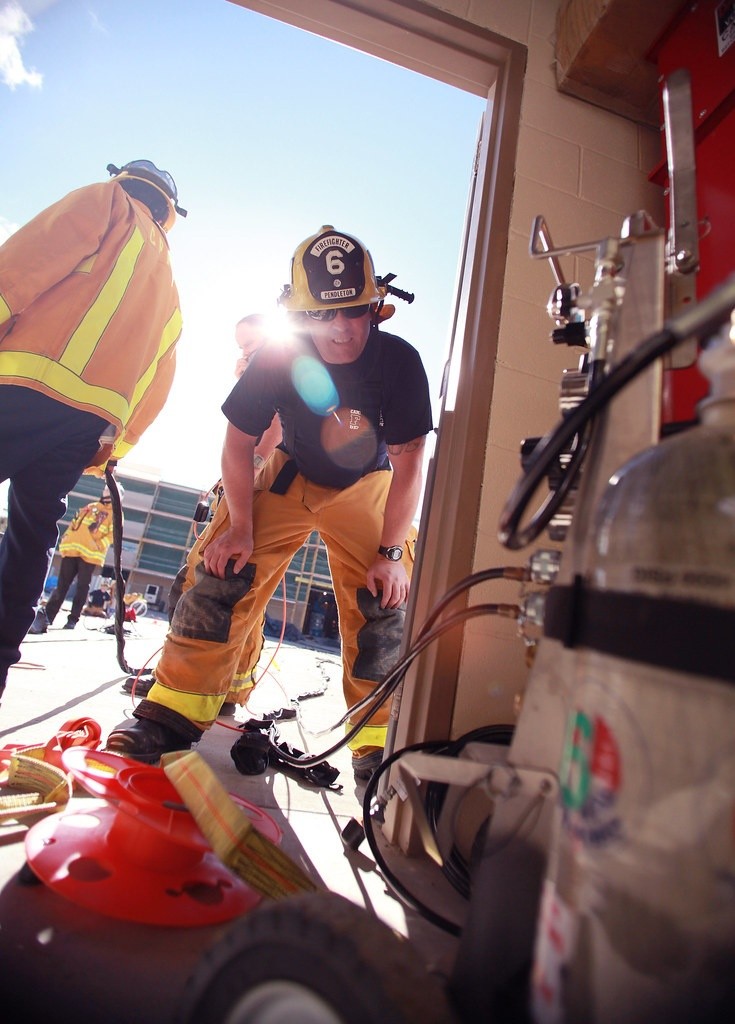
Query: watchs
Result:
<box><xmin>378</xmin><ymin>545</ymin><xmax>403</xmax><ymax>562</ymax></box>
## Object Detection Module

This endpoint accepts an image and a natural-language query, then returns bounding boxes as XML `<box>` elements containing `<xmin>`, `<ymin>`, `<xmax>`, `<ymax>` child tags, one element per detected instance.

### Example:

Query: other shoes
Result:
<box><xmin>218</xmin><ymin>703</ymin><xmax>236</xmax><ymax>716</ymax></box>
<box><xmin>108</xmin><ymin>717</ymin><xmax>192</xmax><ymax>765</ymax></box>
<box><xmin>121</xmin><ymin>676</ymin><xmax>156</xmax><ymax>697</ymax></box>
<box><xmin>63</xmin><ymin>620</ymin><xmax>75</xmax><ymax>629</ymax></box>
<box><xmin>353</xmin><ymin>762</ymin><xmax>380</xmax><ymax>794</ymax></box>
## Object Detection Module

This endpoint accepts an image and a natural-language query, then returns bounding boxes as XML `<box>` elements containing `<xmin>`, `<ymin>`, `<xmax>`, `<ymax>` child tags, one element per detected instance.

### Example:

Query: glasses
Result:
<box><xmin>307</xmin><ymin>303</ymin><xmax>370</xmax><ymax>321</ymax></box>
<box><xmin>116</xmin><ymin>160</ymin><xmax>177</xmax><ymax>200</ymax></box>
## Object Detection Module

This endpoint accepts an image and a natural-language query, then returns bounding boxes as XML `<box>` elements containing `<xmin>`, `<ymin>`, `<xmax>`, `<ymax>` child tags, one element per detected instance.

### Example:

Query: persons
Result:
<box><xmin>106</xmin><ymin>225</ymin><xmax>433</xmax><ymax>781</ymax></box>
<box><xmin>0</xmin><ymin>160</ymin><xmax>188</xmax><ymax>700</ymax></box>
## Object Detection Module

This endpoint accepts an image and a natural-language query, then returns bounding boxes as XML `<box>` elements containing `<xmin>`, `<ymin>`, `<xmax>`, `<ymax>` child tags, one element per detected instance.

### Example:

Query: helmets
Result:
<box><xmin>109</xmin><ymin>165</ymin><xmax>177</xmax><ymax>232</ymax></box>
<box><xmin>282</xmin><ymin>225</ymin><xmax>383</xmax><ymax>310</ymax></box>
